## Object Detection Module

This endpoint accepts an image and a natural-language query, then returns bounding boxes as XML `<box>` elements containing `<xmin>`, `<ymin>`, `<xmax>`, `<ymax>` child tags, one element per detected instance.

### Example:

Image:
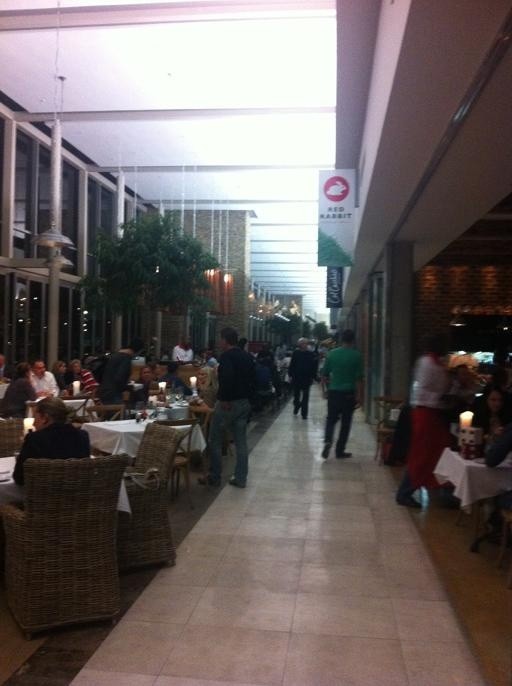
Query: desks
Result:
<box><xmin>432</xmin><ymin>447</ymin><xmax>512</xmax><ymax>554</ymax></box>
<box><xmin>1</xmin><ymin>394</ymin><xmax>208</xmax><ymax>503</ymax></box>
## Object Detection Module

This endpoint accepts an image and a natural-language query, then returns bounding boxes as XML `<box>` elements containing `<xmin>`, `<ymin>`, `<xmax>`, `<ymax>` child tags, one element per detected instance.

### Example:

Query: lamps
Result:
<box><xmin>23</xmin><ymin>376</ymin><xmax>198</xmax><ymax>438</ymax></box>
<box><xmin>458</xmin><ymin>410</ymin><xmax>474</xmax><ymax>428</ymax></box>
<box><xmin>29</xmin><ymin>0</ymin><xmax>77</xmax><ymax>274</ymax></box>
<box><xmin>448</xmin><ymin>309</ymin><xmax>512</xmax><ymax>330</ymax></box>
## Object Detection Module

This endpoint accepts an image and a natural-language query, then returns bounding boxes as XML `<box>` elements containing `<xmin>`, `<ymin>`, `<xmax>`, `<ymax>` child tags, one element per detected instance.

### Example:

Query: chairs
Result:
<box><xmin>373</xmin><ymin>395</ymin><xmax>408</xmax><ymax>468</ymax></box>
<box><xmin>0</xmin><ymin>452</ymin><xmax>132</xmax><ymax>642</ymax></box>
<box><xmin>115</xmin><ymin>423</ymin><xmax>185</xmax><ymax>576</ymax></box>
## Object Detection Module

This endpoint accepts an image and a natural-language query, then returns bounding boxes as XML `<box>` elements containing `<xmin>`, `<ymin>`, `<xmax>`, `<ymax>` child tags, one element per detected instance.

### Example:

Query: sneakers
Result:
<box><xmin>198</xmin><ymin>442</ymin><xmax>421</xmax><ymax>508</ymax></box>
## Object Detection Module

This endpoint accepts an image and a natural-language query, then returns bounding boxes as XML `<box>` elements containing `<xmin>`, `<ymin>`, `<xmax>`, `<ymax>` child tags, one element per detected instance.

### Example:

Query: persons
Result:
<box><xmin>322</xmin><ymin>330</ymin><xmax>365</xmax><ymax>458</ymax></box>
<box><xmin>383</xmin><ymin>332</ymin><xmax>511</xmax><ymax>548</ymax></box>
<box><xmin>13</xmin><ymin>397</ymin><xmax>90</xmax><ymax>485</ymax></box>
<box><xmin>197</xmin><ymin>327</ymin><xmax>257</xmax><ymax>487</ymax></box>
